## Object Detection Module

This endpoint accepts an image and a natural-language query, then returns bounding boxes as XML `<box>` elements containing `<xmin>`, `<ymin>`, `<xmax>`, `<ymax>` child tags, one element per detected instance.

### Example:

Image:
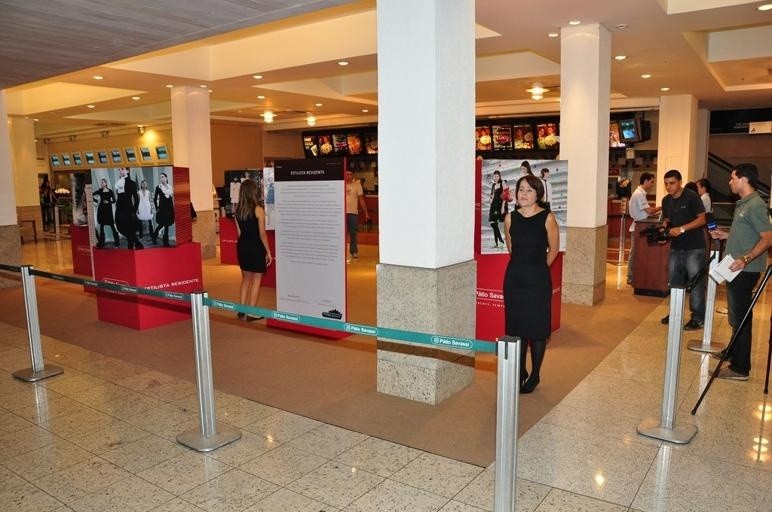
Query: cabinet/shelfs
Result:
<box><xmin>631</xmin><ymin>215</ymin><xmax>672</xmax><ymax>298</ymax></box>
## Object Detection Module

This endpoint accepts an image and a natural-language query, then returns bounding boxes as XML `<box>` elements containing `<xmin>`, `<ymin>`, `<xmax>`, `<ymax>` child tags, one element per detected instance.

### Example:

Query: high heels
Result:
<box><xmin>519</xmin><ymin>370</ymin><xmax>529</xmax><ymax>386</ymax></box>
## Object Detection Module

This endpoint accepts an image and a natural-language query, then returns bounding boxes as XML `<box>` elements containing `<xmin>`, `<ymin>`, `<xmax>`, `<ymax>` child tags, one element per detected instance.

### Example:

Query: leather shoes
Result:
<box><xmin>237</xmin><ymin>311</ymin><xmax>245</xmax><ymax>318</ymax></box>
<box><xmin>247</xmin><ymin>314</ymin><xmax>264</xmax><ymax>321</ymax></box>
<box><xmin>519</xmin><ymin>375</ymin><xmax>540</xmax><ymax>394</ymax></box>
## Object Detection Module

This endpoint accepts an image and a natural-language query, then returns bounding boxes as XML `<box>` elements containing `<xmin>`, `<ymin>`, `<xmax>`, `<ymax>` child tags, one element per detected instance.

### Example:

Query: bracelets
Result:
<box><xmin>744</xmin><ymin>255</ymin><xmax>752</xmax><ymax>264</ymax></box>
<box><xmin>680</xmin><ymin>226</ymin><xmax>686</xmax><ymax>234</ymax></box>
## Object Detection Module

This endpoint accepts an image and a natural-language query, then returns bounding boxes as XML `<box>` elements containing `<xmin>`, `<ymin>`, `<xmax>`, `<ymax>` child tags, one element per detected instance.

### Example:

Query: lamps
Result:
<box><xmin>43</xmin><ymin>126</ymin><xmax>149</xmax><ymax>143</ymax></box>
<box><xmin>524</xmin><ymin>81</ymin><xmax>551</xmax><ymax>101</ymax></box>
<box><xmin>309</xmin><ymin>115</ymin><xmax>317</xmax><ymax>128</ymax></box>
<box><xmin>262</xmin><ymin>109</ymin><xmax>276</xmax><ymax>124</ymax></box>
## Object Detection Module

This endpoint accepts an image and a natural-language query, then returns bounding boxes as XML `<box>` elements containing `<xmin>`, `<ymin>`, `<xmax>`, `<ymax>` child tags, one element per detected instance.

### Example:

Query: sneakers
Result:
<box><xmin>711</xmin><ymin>350</ymin><xmax>732</xmax><ymax>360</ymax></box>
<box><xmin>490</xmin><ymin>240</ymin><xmax>504</xmax><ymax>252</ymax></box>
<box><xmin>708</xmin><ymin>366</ymin><xmax>748</xmax><ymax>381</ymax></box>
<box><xmin>352</xmin><ymin>253</ymin><xmax>359</xmax><ymax>258</ymax></box>
<box><xmin>661</xmin><ymin>314</ymin><xmax>669</xmax><ymax>324</ymax></box>
<box><xmin>683</xmin><ymin>318</ymin><xmax>704</xmax><ymax>330</ymax></box>
<box><xmin>627</xmin><ymin>279</ymin><xmax>633</xmax><ymax>284</ymax></box>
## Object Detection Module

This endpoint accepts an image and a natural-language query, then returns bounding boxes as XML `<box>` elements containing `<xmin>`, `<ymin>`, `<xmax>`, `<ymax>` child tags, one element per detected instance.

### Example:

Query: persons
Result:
<box><xmin>488</xmin><ymin>170</ymin><xmax>513</xmax><ymax>248</ymax></box>
<box><xmin>707</xmin><ymin>163</ymin><xmax>772</xmax><ymax>382</ymax></box>
<box><xmin>536</xmin><ymin>126</ymin><xmax>545</xmax><ymax>137</ymax></box>
<box><xmin>539</xmin><ymin>168</ymin><xmax>553</xmax><ymax>211</ymax></box>
<box><xmin>521</xmin><ymin>161</ymin><xmax>534</xmax><ymax>175</ymax></box>
<box><xmin>696</xmin><ymin>179</ymin><xmax>714</xmax><ymax>212</ymax></box>
<box><xmin>345</xmin><ymin>166</ymin><xmax>369</xmax><ymax>258</ymax></box>
<box><xmin>230</xmin><ymin>176</ymin><xmax>241</xmax><ymax>215</ymax></box>
<box><xmin>233</xmin><ymin>180</ymin><xmax>273</xmax><ymax>321</ymax></box>
<box><xmin>503</xmin><ymin>175</ymin><xmax>559</xmax><ymax>394</ymax></box>
<box><xmin>92</xmin><ymin>167</ymin><xmax>175</xmax><ymax>249</ymax></box>
<box><xmin>657</xmin><ymin>170</ymin><xmax>707</xmax><ymax>330</ymax></box>
<box><xmin>626</xmin><ymin>173</ymin><xmax>663</xmax><ymax>285</ymax></box>
<box><xmin>39</xmin><ymin>176</ymin><xmax>58</xmax><ymax>230</ymax></box>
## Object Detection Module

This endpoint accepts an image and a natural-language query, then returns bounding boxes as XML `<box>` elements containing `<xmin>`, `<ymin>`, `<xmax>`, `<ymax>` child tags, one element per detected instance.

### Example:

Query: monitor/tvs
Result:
<box><xmin>49</xmin><ymin>143</ymin><xmax>171</xmax><ymax>168</ymax></box>
<box><xmin>618</xmin><ymin>118</ymin><xmax>642</xmax><ymax>143</ymax></box>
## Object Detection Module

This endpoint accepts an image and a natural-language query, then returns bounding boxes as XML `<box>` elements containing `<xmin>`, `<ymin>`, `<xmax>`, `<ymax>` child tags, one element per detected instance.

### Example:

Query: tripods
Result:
<box><xmin>691</xmin><ymin>263</ymin><xmax>772</xmax><ymax>415</ymax></box>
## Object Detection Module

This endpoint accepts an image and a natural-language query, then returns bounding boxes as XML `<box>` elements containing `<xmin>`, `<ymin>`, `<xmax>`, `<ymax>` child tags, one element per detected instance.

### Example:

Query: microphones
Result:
<box><xmin>704</xmin><ymin>212</ymin><xmax>721</xmax><ymax>245</ymax></box>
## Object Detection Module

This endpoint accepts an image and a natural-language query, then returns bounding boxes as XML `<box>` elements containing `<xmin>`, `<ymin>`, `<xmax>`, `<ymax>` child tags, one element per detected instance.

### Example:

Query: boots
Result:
<box><xmin>139</xmin><ymin>226</ymin><xmax>144</xmax><ymax>239</ymax></box>
<box><xmin>152</xmin><ymin>232</ymin><xmax>160</xmax><ymax>245</ymax></box>
<box><xmin>149</xmin><ymin>226</ymin><xmax>154</xmax><ymax>238</ymax></box>
<box><xmin>97</xmin><ymin>231</ymin><xmax>105</xmax><ymax>247</ymax></box>
<box><xmin>113</xmin><ymin>232</ymin><xmax>121</xmax><ymax>247</ymax></box>
<box><xmin>127</xmin><ymin>236</ymin><xmax>144</xmax><ymax>249</ymax></box>
<box><xmin>163</xmin><ymin>234</ymin><xmax>171</xmax><ymax>247</ymax></box>
